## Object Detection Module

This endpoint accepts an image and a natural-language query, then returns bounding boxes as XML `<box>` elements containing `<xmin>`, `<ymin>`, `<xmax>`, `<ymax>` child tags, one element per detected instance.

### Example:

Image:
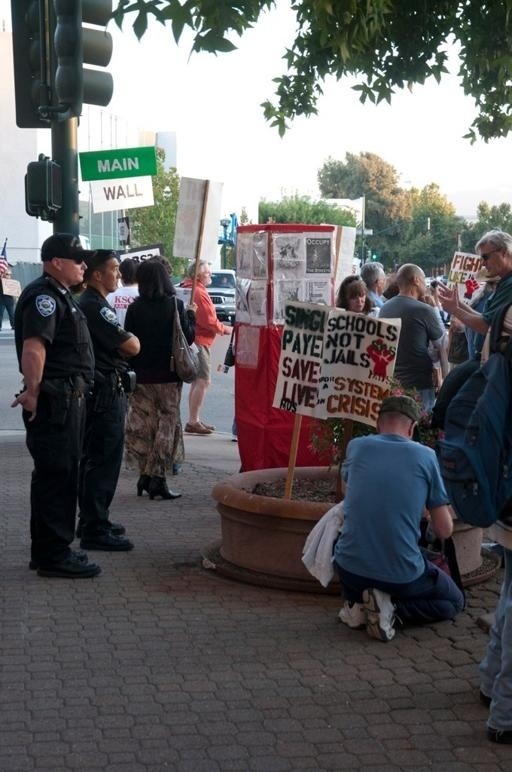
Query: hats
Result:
<box><xmin>378</xmin><ymin>395</ymin><xmax>421</xmax><ymax>441</ymax></box>
<box><xmin>39</xmin><ymin>233</ymin><xmax>97</xmax><ymax>261</ymax></box>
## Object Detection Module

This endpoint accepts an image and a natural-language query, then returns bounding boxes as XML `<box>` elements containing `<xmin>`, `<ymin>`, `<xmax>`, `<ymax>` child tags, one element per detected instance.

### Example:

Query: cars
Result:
<box><xmin>424</xmin><ymin>275</ymin><xmax>448</xmax><ymax>288</ymax></box>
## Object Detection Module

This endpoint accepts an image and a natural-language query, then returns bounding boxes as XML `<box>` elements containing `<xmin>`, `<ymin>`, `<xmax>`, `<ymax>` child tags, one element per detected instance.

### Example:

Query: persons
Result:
<box><xmin>435</xmin><ymin>230</ymin><xmax>511</xmax><ymax>360</ymax></box>
<box><xmin>0</xmin><ymin>278</ymin><xmax>16</xmax><ymax>330</ymax></box>
<box><xmin>10</xmin><ymin>233</ymin><xmax>102</xmax><ymax>580</ymax></box>
<box><xmin>332</xmin><ymin>394</ymin><xmax>465</xmax><ymax>641</ymax></box>
<box><xmin>478</xmin><ymin>340</ymin><xmax>512</xmax><ymax>745</ymax></box>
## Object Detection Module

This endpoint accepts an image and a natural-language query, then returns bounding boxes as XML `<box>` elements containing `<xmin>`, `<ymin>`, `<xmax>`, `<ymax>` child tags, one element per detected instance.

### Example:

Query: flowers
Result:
<box><xmin>306</xmin><ymin>376</ymin><xmax>444</xmax><ymax>473</ymax></box>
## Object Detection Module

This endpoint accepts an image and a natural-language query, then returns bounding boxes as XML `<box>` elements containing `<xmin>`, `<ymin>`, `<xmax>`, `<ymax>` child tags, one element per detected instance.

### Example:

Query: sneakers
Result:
<box><xmin>480</xmin><ymin>683</ymin><xmax>511</xmax><ymax>747</ymax></box>
<box><xmin>364</xmin><ymin>588</ymin><xmax>396</xmax><ymax>642</ymax></box>
<box><xmin>337</xmin><ymin>600</ymin><xmax>366</xmax><ymax>630</ymax></box>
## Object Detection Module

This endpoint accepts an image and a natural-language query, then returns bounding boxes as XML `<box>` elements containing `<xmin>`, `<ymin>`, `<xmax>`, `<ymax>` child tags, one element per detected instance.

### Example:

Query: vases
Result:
<box><xmin>213</xmin><ymin>459</ymin><xmax>486</xmax><ymax>589</ymax></box>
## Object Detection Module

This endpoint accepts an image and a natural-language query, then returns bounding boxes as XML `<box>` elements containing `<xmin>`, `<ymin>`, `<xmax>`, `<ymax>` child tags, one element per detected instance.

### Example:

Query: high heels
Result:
<box><xmin>137</xmin><ymin>472</ymin><xmax>182</xmax><ymax>500</ymax></box>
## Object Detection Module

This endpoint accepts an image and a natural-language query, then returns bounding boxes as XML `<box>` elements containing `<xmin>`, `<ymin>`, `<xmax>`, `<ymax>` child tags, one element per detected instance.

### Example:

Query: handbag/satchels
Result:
<box><xmin>419</xmin><ymin>517</ymin><xmax>450</xmax><ymax>576</ymax></box>
<box><xmin>223</xmin><ymin>342</ymin><xmax>236</xmax><ymax>367</ymax></box>
<box><xmin>448</xmin><ymin>331</ymin><xmax>469</xmax><ymax>363</ymax></box>
<box><xmin>173</xmin><ymin>295</ymin><xmax>199</xmax><ymax>383</ymax></box>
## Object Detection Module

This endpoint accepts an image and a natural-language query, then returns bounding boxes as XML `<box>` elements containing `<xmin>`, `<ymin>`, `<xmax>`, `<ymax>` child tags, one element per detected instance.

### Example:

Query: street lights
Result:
<box><xmin>217</xmin><ymin>214</ymin><xmax>232</xmax><ymax>269</ymax></box>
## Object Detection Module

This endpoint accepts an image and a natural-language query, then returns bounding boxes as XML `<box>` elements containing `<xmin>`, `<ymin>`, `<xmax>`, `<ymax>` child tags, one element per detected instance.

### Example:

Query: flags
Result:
<box><xmin>0</xmin><ymin>241</ymin><xmax>14</xmax><ymax>277</ymax></box>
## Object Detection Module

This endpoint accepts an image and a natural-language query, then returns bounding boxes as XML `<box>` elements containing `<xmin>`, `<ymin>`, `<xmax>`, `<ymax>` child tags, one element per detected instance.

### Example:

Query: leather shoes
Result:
<box><xmin>28</xmin><ymin>550</ymin><xmax>100</xmax><ymax>578</ymax></box>
<box><xmin>79</xmin><ymin>533</ymin><xmax>135</xmax><ymax>551</ymax></box>
<box><xmin>183</xmin><ymin>419</ymin><xmax>215</xmax><ymax>434</ymax></box>
<box><xmin>76</xmin><ymin>522</ymin><xmax>125</xmax><ymax>536</ymax></box>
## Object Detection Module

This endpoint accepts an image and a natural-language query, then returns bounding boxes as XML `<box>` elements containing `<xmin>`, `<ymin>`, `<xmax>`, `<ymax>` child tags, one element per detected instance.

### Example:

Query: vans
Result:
<box><xmin>202</xmin><ymin>267</ymin><xmax>239</xmax><ymax>323</ymax></box>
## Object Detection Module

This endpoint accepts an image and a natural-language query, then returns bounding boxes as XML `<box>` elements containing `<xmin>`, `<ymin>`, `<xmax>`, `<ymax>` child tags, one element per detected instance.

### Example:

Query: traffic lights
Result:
<box><xmin>14</xmin><ymin>0</ymin><xmax>116</xmax><ymax>129</ymax></box>
<box><xmin>24</xmin><ymin>157</ymin><xmax>63</xmax><ymax>209</ymax></box>
<box><xmin>366</xmin><ymin>248</ymin><xmax>371</xmax><ymax>258</ymax></box>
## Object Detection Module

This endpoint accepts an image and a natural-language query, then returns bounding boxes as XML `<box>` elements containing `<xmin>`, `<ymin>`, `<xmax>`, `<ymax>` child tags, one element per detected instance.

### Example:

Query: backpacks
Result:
<box><xmin>429</xmin><ymin>357</ymin><xmax>507</xmax><ymax>529</ymax></box>
